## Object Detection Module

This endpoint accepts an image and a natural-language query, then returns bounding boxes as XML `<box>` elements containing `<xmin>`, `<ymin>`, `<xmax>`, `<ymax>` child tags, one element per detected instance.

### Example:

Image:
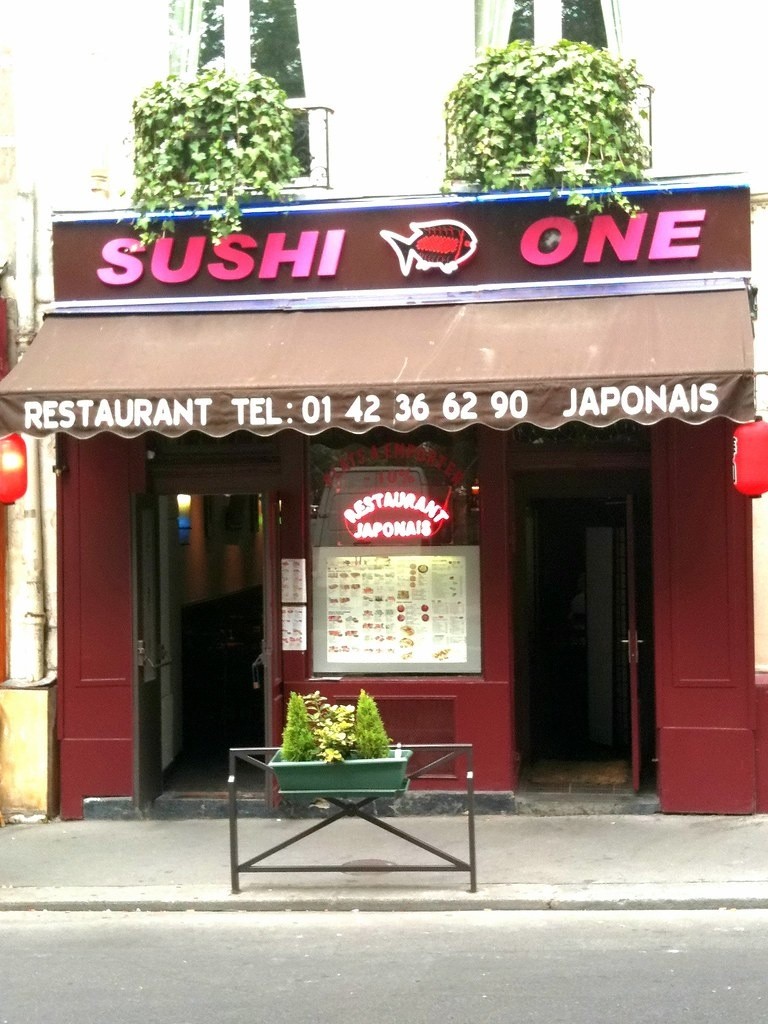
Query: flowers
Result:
<box><xmin>279</xmin><ymin>687</ymin><xmax>395</xmax><ymax>761</ymax></box>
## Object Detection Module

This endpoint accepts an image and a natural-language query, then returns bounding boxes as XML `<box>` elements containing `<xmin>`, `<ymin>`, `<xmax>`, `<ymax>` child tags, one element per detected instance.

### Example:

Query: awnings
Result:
<box><xmin>0</xmin><ymin>289</ymin><xmax>753</xmax><ymax>440</ymax></box>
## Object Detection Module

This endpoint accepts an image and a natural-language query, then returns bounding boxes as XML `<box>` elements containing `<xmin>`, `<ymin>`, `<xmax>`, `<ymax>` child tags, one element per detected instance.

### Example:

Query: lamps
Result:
<box><xmin>178</xmin><ymin>494</ymin><xmax>192</xmax><ymax>545</ymax></box>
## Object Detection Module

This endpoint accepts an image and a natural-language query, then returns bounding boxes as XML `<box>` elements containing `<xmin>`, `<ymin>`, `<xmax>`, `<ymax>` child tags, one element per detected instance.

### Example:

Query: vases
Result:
<box><xmin>267</xmin><ymin>748</ymin><xmax>408</xmax><ymax>795</ymax></box>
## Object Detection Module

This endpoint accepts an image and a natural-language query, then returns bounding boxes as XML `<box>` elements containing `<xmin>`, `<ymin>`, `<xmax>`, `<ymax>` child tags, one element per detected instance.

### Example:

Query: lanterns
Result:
<box><xmin>0</xmin><ymin>433</ymin><xmax>29</xmax><ymax>505</ymax></box>
<box><xmin>731</xmin><ymin>416</ymin><xmax>768</xmax><ymax>499</ymax></box>
